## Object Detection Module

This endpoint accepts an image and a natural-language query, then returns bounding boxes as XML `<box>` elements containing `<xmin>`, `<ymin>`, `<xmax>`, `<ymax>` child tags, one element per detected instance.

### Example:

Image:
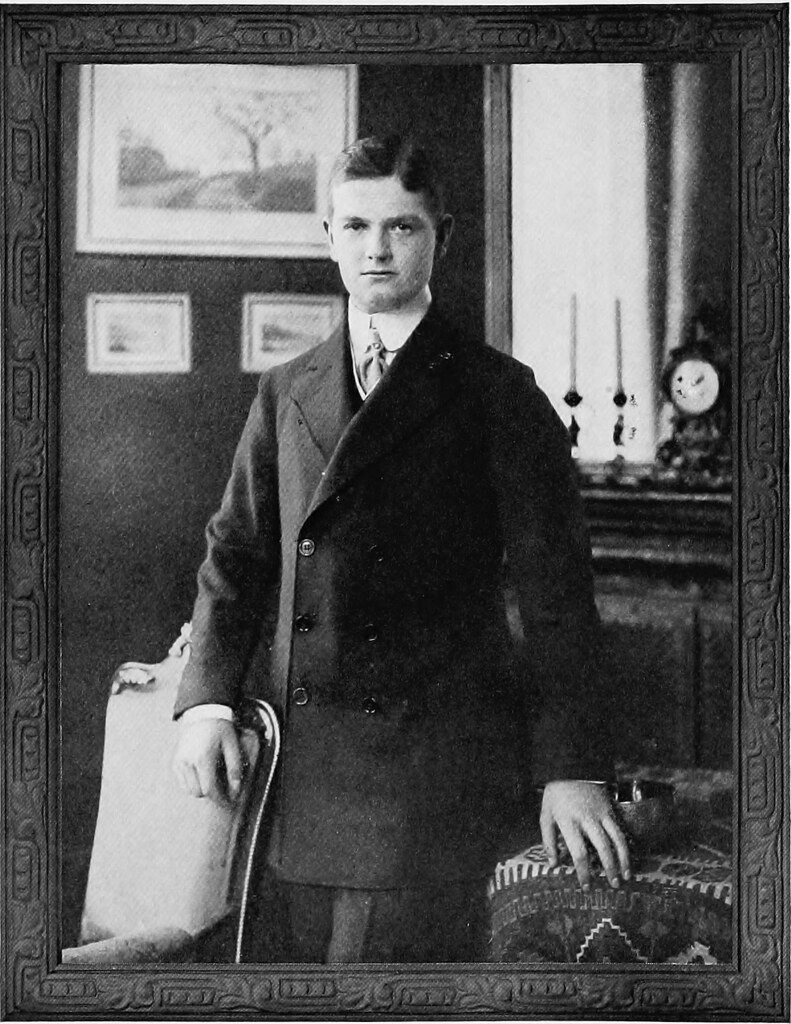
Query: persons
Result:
<box><xmin>173</xmin><ymin>128</ymin><xmax>634</xmax><ymax>966</ymax></box>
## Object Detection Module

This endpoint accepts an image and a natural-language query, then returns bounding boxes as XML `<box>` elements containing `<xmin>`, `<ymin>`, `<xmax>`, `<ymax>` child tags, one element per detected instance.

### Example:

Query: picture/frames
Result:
<box><xmin>75</xmin><ymin>64</ymin><xmax>359</xmax><ymax>262</ymax></box>
<box><xmin>239</xmin><ymin>293</ymin><xmax>347</xmax><ymax>375</ymax></box>
<box><xmin>86</xmin><ymin>288</ymin><xmax>193</xmax><ymax>378</ymax></box>
<box><xmin>0</xmin><ymin>4</ymin><xmax>790</xmax><ymax>1022</ymax></box>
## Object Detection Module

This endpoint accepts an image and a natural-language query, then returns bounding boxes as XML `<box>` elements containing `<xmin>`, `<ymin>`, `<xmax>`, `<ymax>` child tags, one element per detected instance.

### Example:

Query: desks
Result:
<box><xmin>491</xmin><ymin>766</ymin><xmax>732</xmax><ymax>969</ymax></box>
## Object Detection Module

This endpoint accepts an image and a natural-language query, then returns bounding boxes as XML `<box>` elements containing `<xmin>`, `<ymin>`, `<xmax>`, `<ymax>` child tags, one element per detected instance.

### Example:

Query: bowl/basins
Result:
<box><xmin>607</xmin><ymin>780</ymin><xmax>674</xmax><ymax>852</ymax></box>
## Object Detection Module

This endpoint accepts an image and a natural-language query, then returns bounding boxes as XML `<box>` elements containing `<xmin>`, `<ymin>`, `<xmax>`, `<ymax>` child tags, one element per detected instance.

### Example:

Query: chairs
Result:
<box><xmin>60</xmin><ymin>619</ymin><xmax>283</xmax><ymax>964</ymax></box>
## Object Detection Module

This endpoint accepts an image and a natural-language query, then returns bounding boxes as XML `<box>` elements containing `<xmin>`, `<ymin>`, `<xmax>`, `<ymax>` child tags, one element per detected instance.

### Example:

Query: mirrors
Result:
<box><xmin>482</xmin><ymin>65</ymin><xmax>737</xmax><ymax>505</ymax></box>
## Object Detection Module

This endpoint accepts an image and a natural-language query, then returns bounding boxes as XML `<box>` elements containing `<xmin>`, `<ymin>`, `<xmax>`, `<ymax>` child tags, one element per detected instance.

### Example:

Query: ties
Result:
<box><xmin>357</xmin><ymin>326</ymin><xmax>388</xmax><ymax>394</ymax></box>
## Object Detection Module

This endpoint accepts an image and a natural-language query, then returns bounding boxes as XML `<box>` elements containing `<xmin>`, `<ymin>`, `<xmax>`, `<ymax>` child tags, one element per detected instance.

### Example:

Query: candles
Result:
<box><xmin>613</xmin><ymin>295</ymin><xmax>625</xmax><ymax>388</ymax></box>
<box><xmin>567</xmin><ymin>290</ymin><xmax>578</xmax><ymax>394</ymax></box>
